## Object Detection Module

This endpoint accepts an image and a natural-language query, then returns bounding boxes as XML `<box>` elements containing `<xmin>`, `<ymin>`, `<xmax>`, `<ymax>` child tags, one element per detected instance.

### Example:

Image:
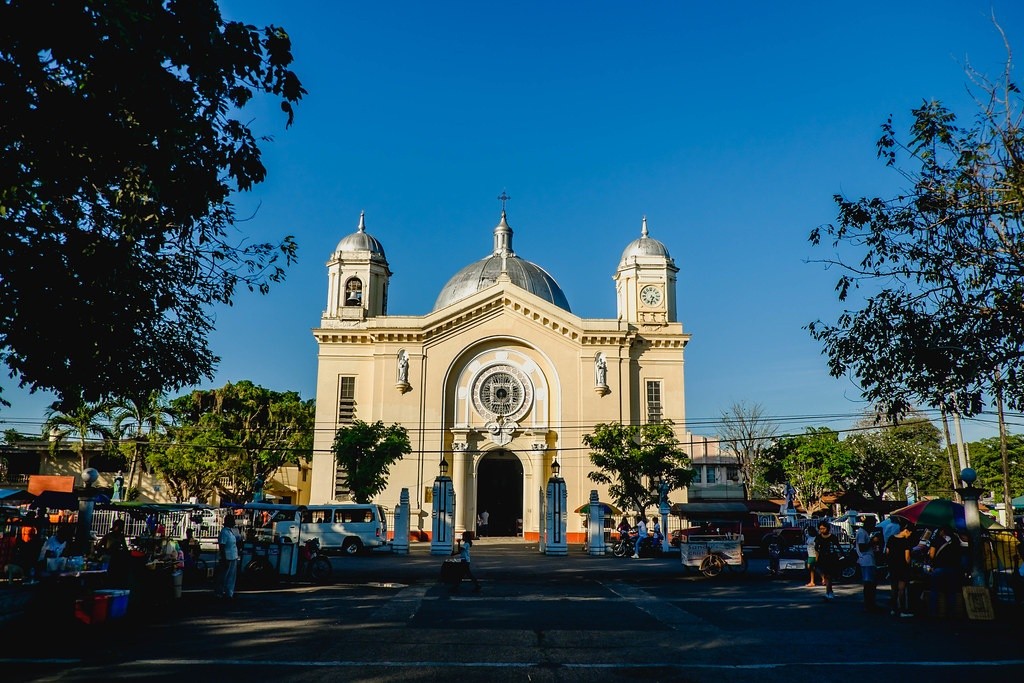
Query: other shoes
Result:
<box><xmin>806</xmin><ymin>583</ymin><xmax>815</xmax><ymax>587</ymax></box>
<box><xmin>901</xmin><ymin>609</ymin><xmax>914</xmax><ymax>617</ymax></box>
<box><xmin>890</xmin><ymin>609</ymin><xmax>897</xmax><ymax>615</ymax></box>
<box><xmin>823</xmin><ymin>591</ymin><xmax>834</xmax><ymax>600</ymax></box>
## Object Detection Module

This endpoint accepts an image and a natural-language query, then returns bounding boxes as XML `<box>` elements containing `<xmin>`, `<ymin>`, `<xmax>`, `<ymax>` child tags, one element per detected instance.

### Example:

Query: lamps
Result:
<box><xmin>640</xmin><ymin>285</ymin><xmax>663</xmax><ymax>307</ymax></box>
<box><xmin>439</xmin><ymin>456</ymin><xmax>448</xmax><ymax>477</ymax></box>
<box><xmin>551</xmin><ymin>458</ymin><xmax>560</xmax><ymax>478</ymax></box>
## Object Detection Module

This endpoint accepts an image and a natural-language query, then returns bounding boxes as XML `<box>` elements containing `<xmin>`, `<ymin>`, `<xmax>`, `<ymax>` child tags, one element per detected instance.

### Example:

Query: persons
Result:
<box><xmin>597</xmin><ymin>358</ymin><xmax>606</xmax><ymax>385</ymax></box>
<box><xmin>454</xmin><ymin>532</ymin><xmax>482</xmax><ymax>591</ymax></box>
<box><xmin>180</xmin><ymin>528</ymin><xmax>200</xmax><ymax>562</ymax></box>
<box><xmin>112</xmin><ymin>470</ymin><xmax>124</xmax><ymax>499</ymax></box>
<box><xmin>0</xmin><ymin>508</ymin><xmax>128</xmax><ymax>578</ymax></box>
<box><xmin>906</xmin><ymin>482</ymin><xmax>915</xmax><ymax>505</ymax></box>
<box><xmin>481</xmin><ymin>509</ymin><xmax>489</xmax><ymax>537</ymax></box>
<box><xmin>254</xmin><ymin>462</ymin><xmax>267</xmax><ymax>501</ymax></box>
<box><xmin>650</xmin><ymin>517</ymin><xmax>660</xmax><ymax>548</ymax></box>
<box><xmin>218</xmin><ymin>514</ymin><xmax>239</xmax><ymax>599</ymax></box>
<box><xmin>398</xmin><ymin>356</ymin><xmax>408</xmax><ymax>380</ymax></box>
<box><xmin>855</xmin><ymin>516</ymin><xmax>972</xmax><ymax>618</ymax></box>
<box><xmin>617</xmin><ymin>516</ymin><xmax>631</xmax><ymax>534</ymax></box>
<box><xmin>814</xmin><ymin>520</ymin><xmax>844</xmax><ymax>600</ymax></box>
<box><xmin>631</xmin><ymin>516</ymin><xmax>647</xmax><ymax>558</ymax></box>
<box><xmin>805</xmin><ymin>526</ymin><xmax>827</xmax><ymax>587</ymax></box>
<box><xmin>781</xmin><ymin>481</ymin><xmax>796</xmax><ymax>509</ymax></box>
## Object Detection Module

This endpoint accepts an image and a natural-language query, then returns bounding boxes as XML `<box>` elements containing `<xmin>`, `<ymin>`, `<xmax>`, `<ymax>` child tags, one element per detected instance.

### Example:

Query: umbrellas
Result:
<box><xmin>1011</xmin><ymin>495</ymin><xmax>1024</xmax><ymax>513</ymax></box>
<box><xmin>890</xmin><ymin>498</ymin><xmax>1005</xmax><ymax>538</ymax></box>
<box><xmin>574</xmin><ymin>501</ymin><xmax>623</xmax><ymax>516</ymax></box>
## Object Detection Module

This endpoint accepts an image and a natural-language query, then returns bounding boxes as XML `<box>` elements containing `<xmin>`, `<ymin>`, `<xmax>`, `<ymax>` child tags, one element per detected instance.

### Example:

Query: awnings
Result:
<box><xmin>242</xmin><ymin>502</ymin><xmax>308</xmax><ymax>542</ymax></box>
<box><xmin>95</xmin><ymin>501</ymin><xmax>217</xmax><ymax>535</ymax></box>
<box><xmin>0</xmin><ymin>488</ymin><xmax>37</xmax><ymax>509</ymax></box>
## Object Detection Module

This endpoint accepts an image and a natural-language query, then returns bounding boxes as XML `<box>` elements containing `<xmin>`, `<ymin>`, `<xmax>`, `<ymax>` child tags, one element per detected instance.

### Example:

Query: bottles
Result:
<box><xmin>82</xmin><ymin>554</ymin><xmax>88</xmax><ymax>571</ymax></box>
<box><xmin>172</xmin><ymin>548</ymin><xmax>184</xmax><ymax>561</ymax></box>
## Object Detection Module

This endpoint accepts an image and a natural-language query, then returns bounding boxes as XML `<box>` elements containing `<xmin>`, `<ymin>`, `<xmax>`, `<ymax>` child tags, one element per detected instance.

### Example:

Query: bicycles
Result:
<box><xmin>297</xmin><ymin>539</ymin><xmax>331</xmax><ymax>582</ymax></box>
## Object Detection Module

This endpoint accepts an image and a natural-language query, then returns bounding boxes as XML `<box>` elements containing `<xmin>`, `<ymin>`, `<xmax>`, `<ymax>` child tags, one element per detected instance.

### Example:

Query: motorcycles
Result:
<box><xmin>613</xmin><ymin>530</ymin><xmax>661</xmax><ymax>557</ymax></box>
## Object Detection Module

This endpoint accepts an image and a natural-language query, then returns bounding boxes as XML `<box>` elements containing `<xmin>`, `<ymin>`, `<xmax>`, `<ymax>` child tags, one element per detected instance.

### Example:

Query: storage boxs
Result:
<box><xmin>76</xmin><ymin>587</ymin><xmax>130</xmax><ymax>625</ymax></box>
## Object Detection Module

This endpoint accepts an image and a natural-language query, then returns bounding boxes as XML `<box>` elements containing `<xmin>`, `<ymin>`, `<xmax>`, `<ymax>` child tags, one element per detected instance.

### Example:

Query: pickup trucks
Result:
<box><xmin>671</xmin><ymin>513</ymin><xmax>805</xmax><ymax>560</ymax></box>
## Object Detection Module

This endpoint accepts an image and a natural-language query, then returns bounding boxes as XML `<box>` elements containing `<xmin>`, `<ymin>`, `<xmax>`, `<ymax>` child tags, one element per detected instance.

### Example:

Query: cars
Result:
<box><xmin>828</xmin><ymin>512</ymin><xmax>881</xmax><ymax>542</ymax></box>
<box><xmin>202</xmin><ymin>509</ymin><xmax>223</xmax><ymax>533</ymax></box>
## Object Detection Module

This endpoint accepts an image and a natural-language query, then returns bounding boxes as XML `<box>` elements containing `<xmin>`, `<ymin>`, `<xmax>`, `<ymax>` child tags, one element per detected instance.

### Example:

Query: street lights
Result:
<box><xmin>74</xmin><ymin>468</ymin><xmax>99</xmax><ymax>556</ymax></box>
<box><xmin>953</xmin><ymin>468</ymin><xmax>991</xmax><ymax>610</ymax></box>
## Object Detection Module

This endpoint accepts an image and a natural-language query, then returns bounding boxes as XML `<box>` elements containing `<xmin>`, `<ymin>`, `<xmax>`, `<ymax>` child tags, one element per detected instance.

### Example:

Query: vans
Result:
<box><xmin>264</xmin><ymin>504</ymin><xmax>387</xmax><ymax>557</ymax></box>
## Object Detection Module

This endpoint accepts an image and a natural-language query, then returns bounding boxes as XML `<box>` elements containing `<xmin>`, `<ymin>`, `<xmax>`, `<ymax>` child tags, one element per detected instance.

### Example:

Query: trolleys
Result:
<box><xmin>680</xmin><ymin>520</ymin><xmax>747</xmax><ymax>579</ymax></box>
